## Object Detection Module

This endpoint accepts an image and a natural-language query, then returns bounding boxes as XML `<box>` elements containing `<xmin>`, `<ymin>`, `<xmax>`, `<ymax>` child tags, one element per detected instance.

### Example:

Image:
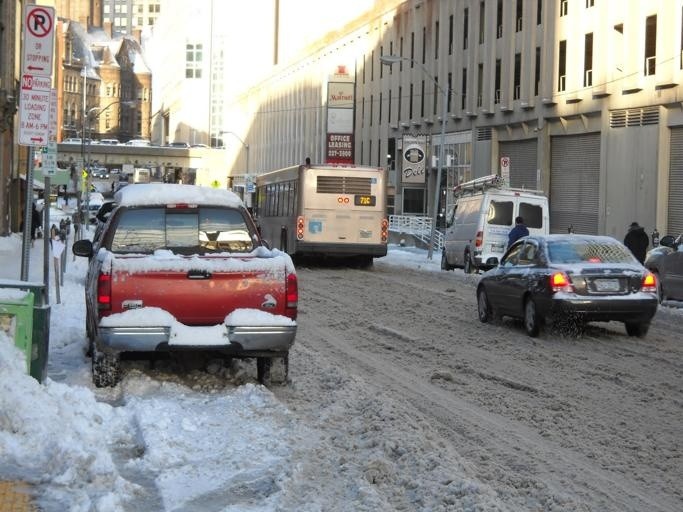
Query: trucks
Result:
<box><xmin>125</xmin><ymin>140</ymin><xmax>150</xmax><ymax>146</ymax></box>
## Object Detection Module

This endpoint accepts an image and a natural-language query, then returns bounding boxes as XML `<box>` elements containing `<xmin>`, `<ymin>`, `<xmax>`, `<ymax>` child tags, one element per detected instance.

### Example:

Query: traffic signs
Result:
<box><xmin>501</xmin><ymin>156</ymin><xmax>511</xmax><ymax>183</ymax></box>
<box><xmin>18</xmin><ymin>75</ymin><xmax>51</xmax><ymax>147</ymax></box>
<box><xmin>20</xmin><ymin>5</ymin><xmax>55</xmax><ymax>75</ymax></box>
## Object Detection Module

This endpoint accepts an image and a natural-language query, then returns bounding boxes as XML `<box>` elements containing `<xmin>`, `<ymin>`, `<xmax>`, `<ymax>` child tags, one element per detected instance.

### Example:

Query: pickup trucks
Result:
<box><xmin>72</xmin><ymin>183</ymin><xmax>298</xmax><ymax>387</ymax></box>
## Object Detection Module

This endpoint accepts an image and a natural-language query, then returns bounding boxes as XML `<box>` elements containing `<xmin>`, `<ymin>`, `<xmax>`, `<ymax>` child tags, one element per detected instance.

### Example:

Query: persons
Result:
<box><xmin>651</xmin><ymin>228</ymin><xmax>659</xmax><ymax>247</ymax></box>
<box><xmin>506</xmin><ymin>217</ymin><xmax>530</xmax><ymax>250</ymax></box>
<box><xmin>32</xmin><ymin>202</ymin><xmax>41</xmax><ymax>240</ymax></box>
<box><xmin>51</xmin><ymin>224</ymin><xmax>58</xmax><ymax>239</ymax></box>
<box><xmin>624</xmin><ymin>221</ymin><xmax>649</xmax><ymax>267</ymax></box>
<box><xmin>60</xmin><ymin>218</ymin><xmax>66</xmax><ymax>233</ymax></box>
<box><xmin>64</xmin><ymin>216</ymin><xmax>71</xmax><ymax>234</ymax></box>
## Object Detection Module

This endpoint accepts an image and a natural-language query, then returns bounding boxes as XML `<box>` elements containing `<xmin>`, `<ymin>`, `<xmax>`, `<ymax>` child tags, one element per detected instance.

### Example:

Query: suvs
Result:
<box><xmin>77</xmin><ymin>201</ymin><xmax>102</xmax><ymax>224</ymax></box>
<box><xmin>159</xmin><ymin>142</ymin><xmax>190</xmax><ymax>149</ymax></box>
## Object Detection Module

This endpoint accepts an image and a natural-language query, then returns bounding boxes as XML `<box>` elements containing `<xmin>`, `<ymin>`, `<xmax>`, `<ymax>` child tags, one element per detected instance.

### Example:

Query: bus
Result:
<box><xmin>128</xmin><ymin>167</ymin><xmax>150</xmax><ymax>183</ymax></box>
<box><xmin>252</xmin><ymin>158</ymin><xmax>388</xmax><ymax>268</ymax></box>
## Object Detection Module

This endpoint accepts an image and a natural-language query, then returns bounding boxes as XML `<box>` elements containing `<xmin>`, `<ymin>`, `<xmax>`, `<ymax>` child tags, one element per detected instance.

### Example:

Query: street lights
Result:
<box><xmin>378</xmin><ymin>56</ymin><xmax>449</xmax><ymax>259</ymax></box>
<box><xmin>218</xmin><ymin>132</ymin><xmax>253</xmax><ymax>210</ymax></box>
<box><xmin>84</xmin><ymin>101</ymin><xmax>138</xmax><ymax>230</ymax></box>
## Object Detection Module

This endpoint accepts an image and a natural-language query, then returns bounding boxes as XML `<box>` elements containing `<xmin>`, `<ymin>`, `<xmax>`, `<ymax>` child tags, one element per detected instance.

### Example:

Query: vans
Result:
<box><xmin>62</xmin><ymin>137</ymin><xmax>91</xmax><ymax>144</ymax></box>
<box><xmin>441</xmin><ymin>174</ymin><xmax>549</xmax><ymax>273</ymax></box>
<box><xmin>101</xmin><ymin>139</ymin><xmax>119</xmax><ymax>145</ymax></box>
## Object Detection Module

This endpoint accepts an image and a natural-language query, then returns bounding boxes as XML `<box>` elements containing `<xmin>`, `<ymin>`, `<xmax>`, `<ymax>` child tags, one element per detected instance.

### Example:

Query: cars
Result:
<box><xmin>99</xmin><ymin>170</ymin><xmax>109</xmax><ymax>179</ymax></box>
<box><xmin>644</xmin><ymin>233</ymin><xmax>683</xmax><ymax>303</ymax></box>
<box><xmin>477</xmin><ymin>225</ymin><xmax>658</xmax><ymax>336</ymax></box>
<box><xmin>92</xmin><ymin>169</ymin><xmax>100</xmax><ymax>177</ymax></box>
